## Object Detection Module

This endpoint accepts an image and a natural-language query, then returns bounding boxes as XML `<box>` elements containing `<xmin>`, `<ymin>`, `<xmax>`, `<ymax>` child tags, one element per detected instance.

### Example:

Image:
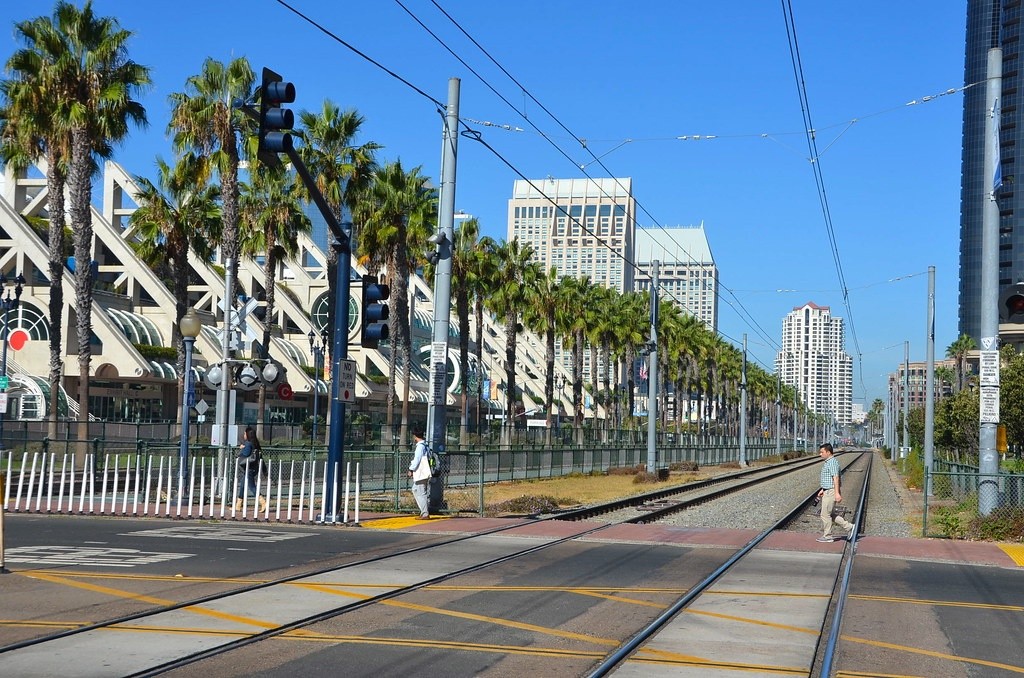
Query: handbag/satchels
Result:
<box><xmin>238</xmin><ymin>442</ymin><xmax>258</xmax><ymax>465</ymax></box>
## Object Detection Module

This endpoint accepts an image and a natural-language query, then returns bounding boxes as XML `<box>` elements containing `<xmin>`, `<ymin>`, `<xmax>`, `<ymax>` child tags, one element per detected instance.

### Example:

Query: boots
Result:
<box><xmin>228</xmin><ymin>497</ymin><xmax>242</xmax><ymax>512</ymax></box>
<box><xmin>259</xmin><ymin>495</ymin><xmax>267</xmax><ymax>513</ymax></box>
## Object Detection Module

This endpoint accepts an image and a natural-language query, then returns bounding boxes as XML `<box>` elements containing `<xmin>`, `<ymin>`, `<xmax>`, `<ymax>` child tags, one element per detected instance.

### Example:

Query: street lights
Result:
<box><xmin>174</xmin><ymin>306</ymin><xmax>202</xmax><ymax>499</ymax></box>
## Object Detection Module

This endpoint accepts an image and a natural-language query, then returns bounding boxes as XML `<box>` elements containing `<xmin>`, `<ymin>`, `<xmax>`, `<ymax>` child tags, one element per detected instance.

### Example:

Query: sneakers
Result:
<box><xmin>846</xmin><ymin>524</ymin><xmax>855</xmax><ymax>540</ymax></box>
<box><xmin>816</xmin><ymin>536</ymin><xmax>833</xmax><ymax>542</ymax></box>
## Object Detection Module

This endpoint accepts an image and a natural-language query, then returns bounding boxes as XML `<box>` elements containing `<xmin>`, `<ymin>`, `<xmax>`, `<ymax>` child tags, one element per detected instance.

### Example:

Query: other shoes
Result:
<box><xmin>415</xmin><ymin>515</ymin><xmax>430</xmax><ymax>520</ymax></box>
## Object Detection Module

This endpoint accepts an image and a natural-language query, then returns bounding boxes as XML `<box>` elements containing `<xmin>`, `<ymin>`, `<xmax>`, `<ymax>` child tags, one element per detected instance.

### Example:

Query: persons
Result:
<box><xmin>229</xmin><ymin>427</ymin><xmax>266</xmax><ymax>512</ymax></box>
<box><xmin>816</xmin><ymin>443</ymin><xmax>855</xmax><ymax>542</ymax></box>
<box><xmin>408</xmin><ymin>425</ymin><xmax>432</xmax><ymax>520</ymax></box>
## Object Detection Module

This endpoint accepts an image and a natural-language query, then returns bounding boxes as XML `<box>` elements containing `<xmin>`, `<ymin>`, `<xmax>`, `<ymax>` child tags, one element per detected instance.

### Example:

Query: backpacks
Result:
<box><xmin>420</xmin><ymin>443</ymin><xmax>442</xmax><ymax>477</ymax></box>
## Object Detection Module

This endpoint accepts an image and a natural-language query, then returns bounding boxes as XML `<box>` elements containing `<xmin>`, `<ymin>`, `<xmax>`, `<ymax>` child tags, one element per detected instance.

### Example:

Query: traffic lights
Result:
<box><xmin>360</xmin><ymin>274</ymin><xmax>390</xmax><ymax>350</ymax></box>
